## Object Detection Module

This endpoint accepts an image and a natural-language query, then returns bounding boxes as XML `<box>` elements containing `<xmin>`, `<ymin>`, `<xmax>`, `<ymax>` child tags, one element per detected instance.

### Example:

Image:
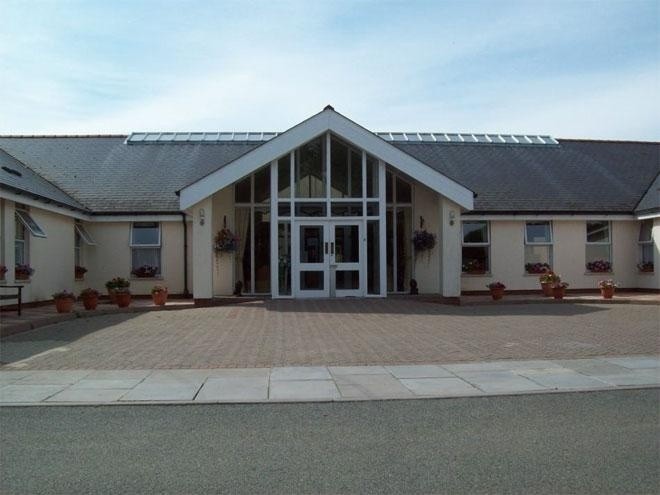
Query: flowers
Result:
<box><xmin>411</xmin><ymin>230</ymin><xmax>655</xmax><ymax>290</ymax></box>
<box><xmin>0</xmin><ymin>230</ymin><xmax>243</xmax><ymax>300</ymax></box>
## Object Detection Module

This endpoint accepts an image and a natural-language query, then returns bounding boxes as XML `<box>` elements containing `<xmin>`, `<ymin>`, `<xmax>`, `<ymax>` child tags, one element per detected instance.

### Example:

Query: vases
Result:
<box><xmin>489</xmin><ymin>282</ymin><xmax>615</xmax><ymax>300</ymax></box>
<box><xmin>56</xmin><ymin>289</ymin><xmax>168</xmax><ymax>313</ymax></box>
